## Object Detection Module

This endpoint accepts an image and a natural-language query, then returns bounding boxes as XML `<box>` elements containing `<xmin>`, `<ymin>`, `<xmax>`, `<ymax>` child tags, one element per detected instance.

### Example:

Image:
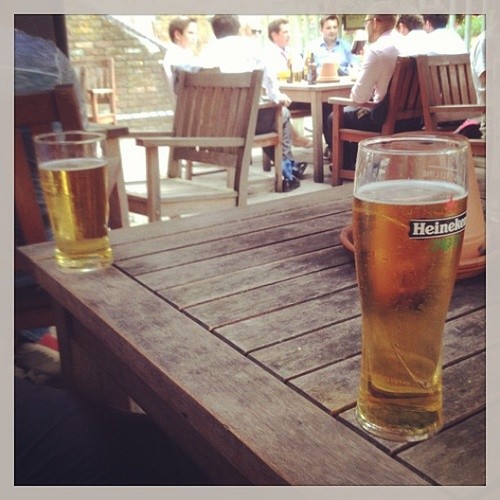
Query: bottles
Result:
<box><xmin>307</xmin><ymin>53</ymin><xmax>318</xmax><ymax>86</ymax></box>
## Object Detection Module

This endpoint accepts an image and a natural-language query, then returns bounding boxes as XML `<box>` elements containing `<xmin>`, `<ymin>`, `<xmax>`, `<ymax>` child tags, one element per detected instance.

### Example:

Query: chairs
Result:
<box><xmin>326</xmin><ymin>54</ymin><xmax>422</xmax><ymax>188</ymax></box>
<box><xmin>415</xmin><ymin>53</ymin><xmax>486</xmax><ymax>160</ymax></box>
<box><xmin>252</xmin><ymin>100</ymin><xmax>284</xmax><ymax>192</ymax></box>
<box><xmin>80</xmin><ymin>55</ymin><xmax>118</xmax><ymax>122</ymax></box>
<box><xmin>14</xmin><ymin>80</ymin><xmax>129</xmax><ymax>243</ymax></box>
<box><xmin>127</xmin><ymin>68</ymin><xmax>265</xmax><ymax>226</ymax></box>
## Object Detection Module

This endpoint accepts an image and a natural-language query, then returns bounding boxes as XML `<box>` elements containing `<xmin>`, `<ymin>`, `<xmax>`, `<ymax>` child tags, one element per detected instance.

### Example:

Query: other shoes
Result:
<box><xmin>293</xmin><ymin>162</ymin><xmax>307</xmax><ymax>178</ymax></box>
<box><xmin>282</xmin><ymin>178</ymin><xmax>300</xmax><ymax>191</ymax></box>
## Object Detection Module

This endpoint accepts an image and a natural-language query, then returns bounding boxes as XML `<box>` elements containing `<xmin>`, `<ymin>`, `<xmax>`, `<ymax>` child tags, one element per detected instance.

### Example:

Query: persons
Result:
<box><xmin>14</xmin><ymin>28</ymin><xmax>90</xmax><ymax>248</ymax></box>
<box><xmin>163</xmin><ymin>14</ymin><xmax>487</xmax><ymax>193</ymax></box>
<box><xmin>14</xmin><ymin>376</ymin><xmax>121</xmax><ymax>486</ymax></box>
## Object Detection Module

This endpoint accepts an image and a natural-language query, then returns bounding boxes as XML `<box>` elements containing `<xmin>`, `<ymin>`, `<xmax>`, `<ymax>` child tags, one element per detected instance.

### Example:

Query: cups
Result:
<box><xmin>33</xmin><ymin>131</ymin><xmax>114</xmax><ymax>272</ymax></box>
<box><xmin>293</xmin><ymin>66</ymin><xmax>304</xmax><ymax>84</ymax></box>
<box><xmin>353</xmin><ymin>131</ymin><xmax>469</xmax><ymax>443</ymax></box>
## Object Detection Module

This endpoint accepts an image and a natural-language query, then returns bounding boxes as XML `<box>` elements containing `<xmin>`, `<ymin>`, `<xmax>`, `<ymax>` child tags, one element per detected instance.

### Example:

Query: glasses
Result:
<box><xmin>362</xmin><ymin>19</ymin><xmax>382</xmax><ymax>24</ymax></box>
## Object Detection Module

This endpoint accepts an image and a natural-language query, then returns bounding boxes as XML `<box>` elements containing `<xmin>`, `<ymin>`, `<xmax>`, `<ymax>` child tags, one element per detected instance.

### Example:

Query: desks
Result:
<box><xmin>276</xmin><ymin>83</ymin><xmax>354</xmax><ymax>182</ymax></box>
<box><xmin>14</xmin><ymin>164</ymin><xmax>486</xmax><ymax>486</ymax></box>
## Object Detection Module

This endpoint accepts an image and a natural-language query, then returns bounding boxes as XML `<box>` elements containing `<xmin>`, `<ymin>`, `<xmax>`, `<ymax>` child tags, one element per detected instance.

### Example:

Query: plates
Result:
<box><xmin>316</xmin><ymin>76</ymin><xmax>340</xmax><ymax>83</ymax></box>
<box><xmin>340</xmin><ymin>224</ymin><xmax>486</xmax><ymax>282</ymax></box>
